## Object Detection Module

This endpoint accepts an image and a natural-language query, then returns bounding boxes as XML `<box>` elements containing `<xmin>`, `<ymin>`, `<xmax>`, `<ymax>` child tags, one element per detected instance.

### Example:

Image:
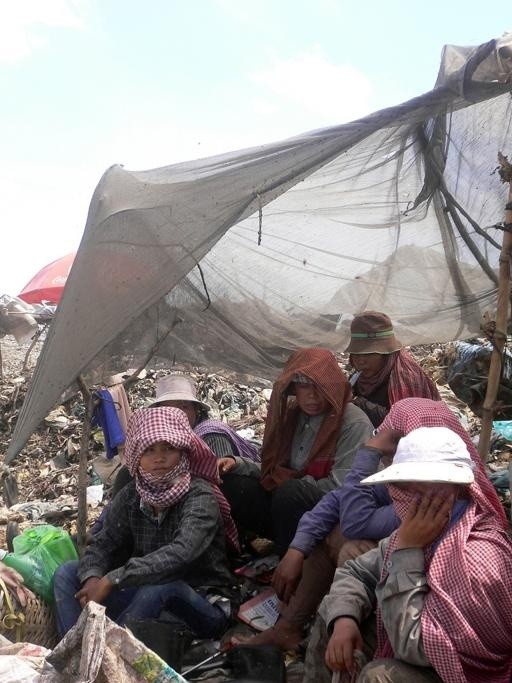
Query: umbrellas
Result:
<box><xmin>16</xmin><ymin>252</ymin><xmax>76</xmax><ymax>306</ymax></box>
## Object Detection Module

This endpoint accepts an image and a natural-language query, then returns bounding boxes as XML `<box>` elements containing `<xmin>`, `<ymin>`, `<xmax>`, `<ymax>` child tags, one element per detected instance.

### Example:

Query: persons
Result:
<box><xmin>230</xmin><ymin>396</ymin><xmax>487</xmax><ymax>653</ymax></box>
<box><xmin>216</xmin><ymin>348</ymin><xmax>374</xmax><ymax>561</ymax></box>
<box><xmin>148</xmin><ymin>373</ymin><xmax>261</xmax><ymax>464</ymax></box>
<box><xmin>344</xmin><ymin>311</ymin><xmax>442</xmax><ymax>427</ymax></box>
<box><xmin>52</xmin><ymin>406</ymin><xmax>242</xmax><ymax>643</ymax></box>
<box><xmin>301</xmin><ymin>428</ymin><xmax>511</xmax><ymax>682</ymax></box>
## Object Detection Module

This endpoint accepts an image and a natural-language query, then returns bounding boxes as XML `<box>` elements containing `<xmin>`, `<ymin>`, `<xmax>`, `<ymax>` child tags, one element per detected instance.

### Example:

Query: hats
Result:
<box><xmin>145</xmin><ymin>375</ymin><xmax>213</xmax><ymax>413</ymax></box>
<box><xmin>359</xmin><ymin>426</ymin><xmax>476</xmax><ymax>487</ymax></box>
<box><xmin>345</xmin><ymin>311</ymin><xmax>405</xmax><ymax>359</ymax></box>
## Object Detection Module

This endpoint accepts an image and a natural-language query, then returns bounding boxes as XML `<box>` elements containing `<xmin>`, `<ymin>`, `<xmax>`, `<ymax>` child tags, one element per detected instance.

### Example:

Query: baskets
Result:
<box><xmin>0</xmin><ymin>585</ymin><xmax>59</xmax><ymax>650</ymax></box>
